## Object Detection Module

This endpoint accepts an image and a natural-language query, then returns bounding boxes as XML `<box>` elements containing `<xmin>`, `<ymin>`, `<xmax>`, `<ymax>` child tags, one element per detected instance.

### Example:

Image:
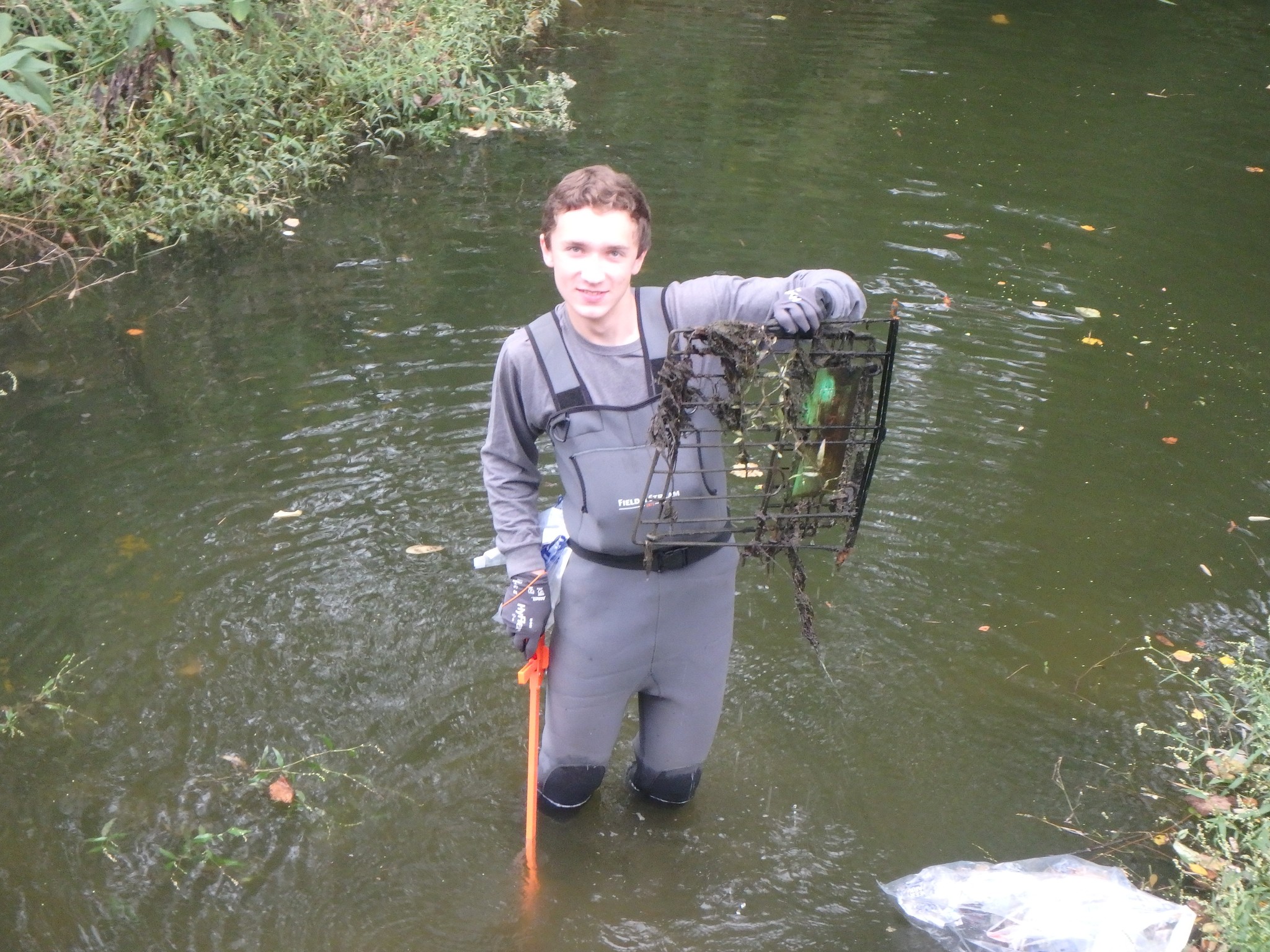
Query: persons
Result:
<box><xmin>480</xmin><ymin>164</ymin><xmax>867</xmax><ymax>822</ymax></box>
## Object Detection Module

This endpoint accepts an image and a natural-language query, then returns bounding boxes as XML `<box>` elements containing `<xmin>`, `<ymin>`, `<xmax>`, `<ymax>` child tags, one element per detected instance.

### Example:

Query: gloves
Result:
<box><xmin>500</xmin><ymin>572</ymin><xmax>552</xmax><ymax>660</ymax></box>
<box><xmin>773</xmin><ymin>287</ymin><xmax>828</xmax><ymax>335</ymax></box>
